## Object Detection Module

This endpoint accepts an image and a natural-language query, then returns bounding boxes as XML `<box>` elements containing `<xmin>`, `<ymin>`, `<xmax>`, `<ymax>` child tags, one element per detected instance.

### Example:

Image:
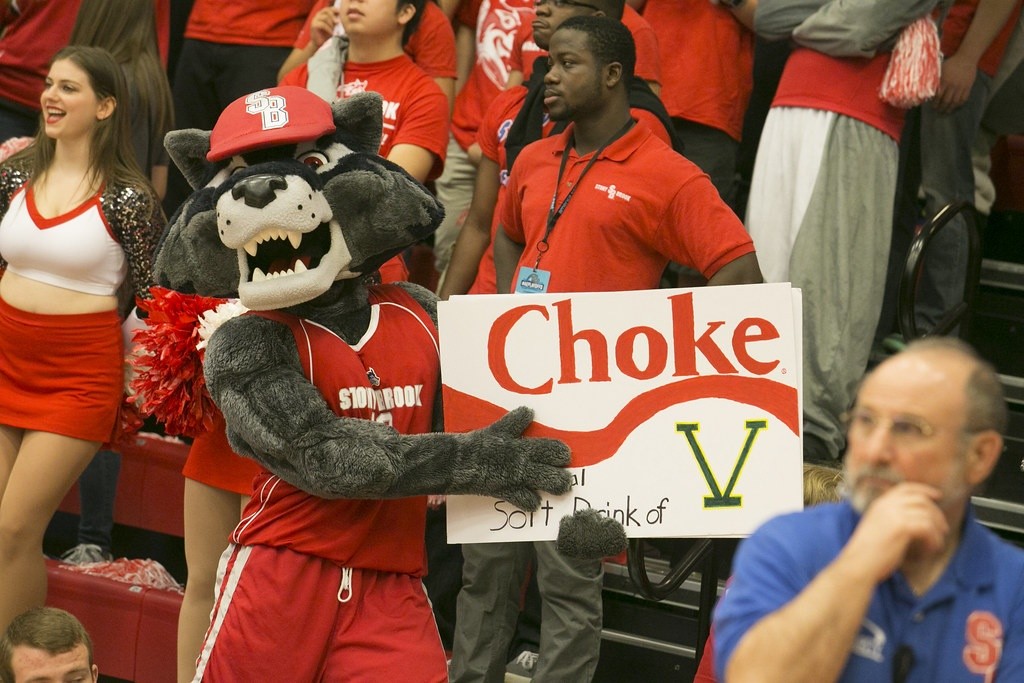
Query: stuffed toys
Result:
<box><xmin>150</xmin><ymin>85</ymin><xmax>628</xmax><ymax>683</ymax></box>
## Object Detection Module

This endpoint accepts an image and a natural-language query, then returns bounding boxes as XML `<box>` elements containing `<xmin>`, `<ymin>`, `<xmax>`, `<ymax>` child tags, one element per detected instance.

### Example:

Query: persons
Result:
<box><xmin>0</xmin><ymin>0</ymin><xmax>1024</xmax><ymax>683</ymax></box>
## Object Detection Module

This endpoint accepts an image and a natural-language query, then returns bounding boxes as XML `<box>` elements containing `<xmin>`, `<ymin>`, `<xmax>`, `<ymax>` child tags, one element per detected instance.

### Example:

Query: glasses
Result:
<box><xmin>839</xmin><ymin>411</ymin><xmax>995</xmax><ymax>449</ymax></box>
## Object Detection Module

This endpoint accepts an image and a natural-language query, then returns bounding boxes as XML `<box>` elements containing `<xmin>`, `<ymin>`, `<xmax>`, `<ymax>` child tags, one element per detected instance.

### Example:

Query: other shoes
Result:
<box><xmin>61</xmin><ymin>542</ymin><xmax>111</xmax><ymax>570</ymax></box>
<box><xmin>882</xmin><ymin>330</ymin><xmax>908</xmax><ymax>354</ymax></box>
<box><xmin>504</xmin><ymin>650</ymin><xmax>539</xmax><ymax>683</ymax></box>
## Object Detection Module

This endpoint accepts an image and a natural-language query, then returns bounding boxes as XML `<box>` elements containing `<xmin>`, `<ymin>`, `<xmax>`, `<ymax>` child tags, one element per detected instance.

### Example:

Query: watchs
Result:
<box><xmin>718</xmin><ymin>0</ymin><xmax>745</xmax><ymax>12</ymax></box>
